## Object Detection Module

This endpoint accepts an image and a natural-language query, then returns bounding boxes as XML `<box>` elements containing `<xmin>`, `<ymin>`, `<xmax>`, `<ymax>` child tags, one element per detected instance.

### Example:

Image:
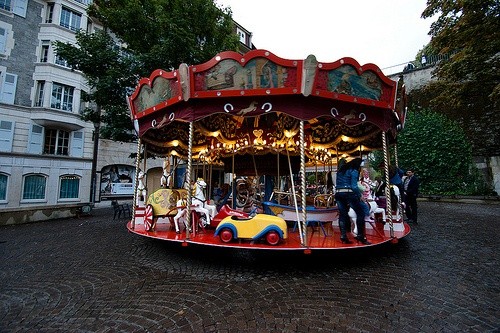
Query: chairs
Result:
<box><xmin>110</xmin><ymin>198</ymin><xmax>131</xmax><ymax>219</ymax></box>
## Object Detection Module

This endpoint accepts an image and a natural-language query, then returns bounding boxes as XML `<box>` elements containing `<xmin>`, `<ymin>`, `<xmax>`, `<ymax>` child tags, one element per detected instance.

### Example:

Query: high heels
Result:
<box><xmin>340</xmin><ymin>237</ymin><xmax>353</xmax><ymax>244</ymax></box>
<box><xmin>357</xmin><ymin>237</ymin><xmax>372</xmax><ymax>244</ymax></box>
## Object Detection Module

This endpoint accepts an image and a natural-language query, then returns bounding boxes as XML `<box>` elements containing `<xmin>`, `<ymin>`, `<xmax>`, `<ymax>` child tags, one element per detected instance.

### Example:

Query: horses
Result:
<box><xmin>136</xmin><ymin>172</ymin><xmax>147</xmax><ymax>206</ymax></box>
<box><xmin>174</xmin><ymin>177</ymin><xmax>217</xmax><ymax>234</ymax></box>
<box><xmin>161</xmin><ymin>160</ymin><xmax>171</xmax><ymax>187</ymax></box>
<box><xmin>347</xmin><ymin>183</ymin><xmax>385</xmax><ymax>238</ymax></box>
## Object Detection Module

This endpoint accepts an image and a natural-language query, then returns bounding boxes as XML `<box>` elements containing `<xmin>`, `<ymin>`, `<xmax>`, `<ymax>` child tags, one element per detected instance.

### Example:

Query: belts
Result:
<box><xmin>336</xmin><ymin>189</ymin><xmax>353</xmax><ymax>192</ymax></box>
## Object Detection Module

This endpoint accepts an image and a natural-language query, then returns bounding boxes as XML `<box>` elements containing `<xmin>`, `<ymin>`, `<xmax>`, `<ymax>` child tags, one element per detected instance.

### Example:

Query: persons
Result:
<box><xmin>384</xmin><ymin>164</ymin><xmax>404</xmax><ymax>211</ymax></box>
<box><xmin>402</xmin><ymin>168</ymin><xmax>418</xmax><ymax>223</ymax></box>
<box><xmin>335</xmin><ymin>158</ymin><xmax>372</xmax><ymax>245</ymax></box>
